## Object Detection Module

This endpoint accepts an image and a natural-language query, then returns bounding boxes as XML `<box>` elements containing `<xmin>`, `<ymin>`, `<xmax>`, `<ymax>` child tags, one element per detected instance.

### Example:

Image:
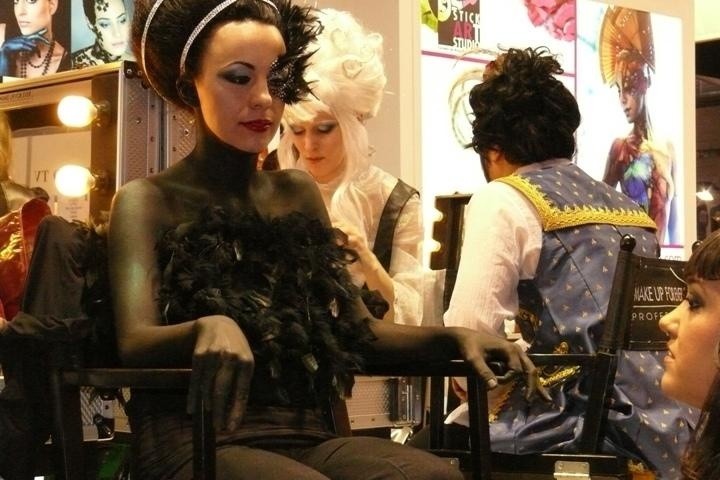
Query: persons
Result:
<box><xmin>71</xmin><ymin>0</ymin><xmax>138</xmax><ymax>70</ymax></box>
<box><xmin>101</xmin><ymin>0</ymin><xmax>554</xmax><ymax>480</ymax></box>
<box><xmin>0</xmin><ymin>0</ymin><xmax>72</xmax><ymax>84</ymax></box>
<box><xmin>265</xmin><ymin>8</ymin><xmax>424</xmax><ymax>440</ymax></box>
<box><xmin>403</xmin><ymin>43</ymin><xmax>709</xmax><ymax>480</ymax></box>
<box><xmin>659</xmin><ymin>228</ymin><xmax>720</xmax><ymax>480</ymax></box>
<box><xmin>602</xmin><ymin>52</ymin><xmax>679</xmax><ymax>246</ymax></box>
<box><xmin>0</xmin><ymin>109</ymin><xmax>53</xmax><ymax>321</ymax></box>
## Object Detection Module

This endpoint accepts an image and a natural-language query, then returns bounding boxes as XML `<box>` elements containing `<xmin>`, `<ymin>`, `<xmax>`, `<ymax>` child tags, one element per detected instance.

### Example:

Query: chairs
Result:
<box><xmin>40</xmin><ymin>232</ymin><xmax>509</xmax><ymax>480</ymax></box>
<box><xmin>422</xmin><ymin>233</ymin><xmax>690</xmax><ymax>480</ymax></box>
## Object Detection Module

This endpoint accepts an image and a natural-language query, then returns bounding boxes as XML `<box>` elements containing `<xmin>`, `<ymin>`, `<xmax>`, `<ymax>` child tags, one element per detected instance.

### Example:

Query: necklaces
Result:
<box><xmin>20</xmin><ymin>35</ymin><xmax>55</xmax><ymax>78</ymax></box>
